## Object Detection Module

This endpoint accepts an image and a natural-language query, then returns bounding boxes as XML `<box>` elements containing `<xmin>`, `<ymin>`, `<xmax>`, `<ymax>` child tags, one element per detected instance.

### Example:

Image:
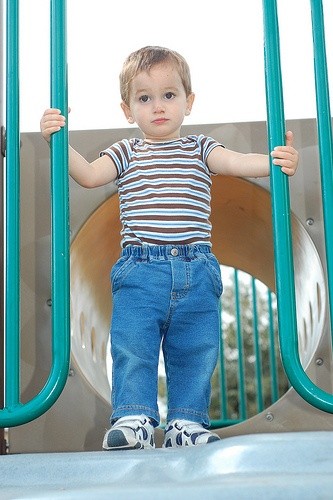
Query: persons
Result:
<box><xmin>39</xmin><ymin>46</ymin><xmax>298</xmax><ymax>449</ymax></box>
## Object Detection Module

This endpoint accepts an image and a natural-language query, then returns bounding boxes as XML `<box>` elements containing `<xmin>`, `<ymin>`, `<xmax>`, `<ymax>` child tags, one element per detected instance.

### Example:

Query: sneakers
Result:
<box><xmin>161</xmin><ymin>418</ymin><xmax>220</xmax><ymax>447</ymax></box>
<box><xmin>102</xmin><ymin>414</ymin><xmax>156</xmax><ymax>450</ymax></box>
